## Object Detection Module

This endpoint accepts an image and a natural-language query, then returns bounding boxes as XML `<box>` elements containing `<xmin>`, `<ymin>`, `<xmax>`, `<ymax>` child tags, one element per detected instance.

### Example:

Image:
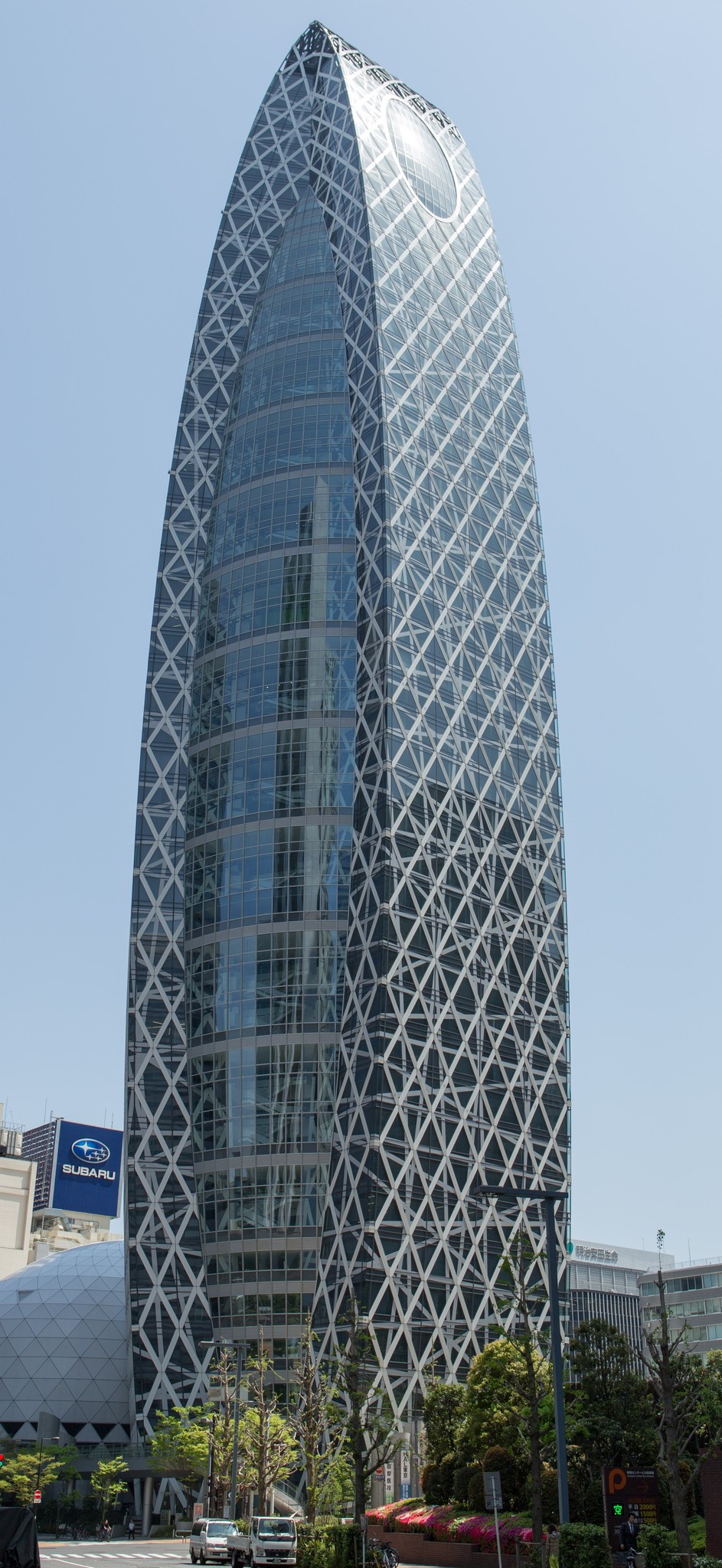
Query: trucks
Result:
<box><xmin>227</xmin><ymin>1516</ymin><xmax>305</xmax><ymax>1568</ymax></box>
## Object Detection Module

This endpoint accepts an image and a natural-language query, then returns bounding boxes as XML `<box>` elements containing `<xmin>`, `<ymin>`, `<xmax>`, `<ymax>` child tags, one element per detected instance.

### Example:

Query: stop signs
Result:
<box><xmin>34</xmin><ymin>1490</ymin><xmax>41</xmax><ymax>1499</ymax></box>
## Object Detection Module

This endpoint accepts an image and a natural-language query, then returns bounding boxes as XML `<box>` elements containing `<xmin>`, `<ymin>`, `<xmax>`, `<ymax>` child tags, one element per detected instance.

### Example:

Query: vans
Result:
<box><xmin>189</xmin><ymin>1518</ymin><xmax>243</xmax><ymax>1565</ymax></box>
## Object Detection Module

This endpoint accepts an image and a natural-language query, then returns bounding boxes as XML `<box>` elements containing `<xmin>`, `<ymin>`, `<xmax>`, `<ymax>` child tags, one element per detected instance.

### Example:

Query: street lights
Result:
<box><xmin>33</xmin><ymin>1437</ymin><xmax>60</xmax><ymax>1522</ymax></box>
<box><xmin>198</xmin><ymin>1341</ymin><xmax>250</xmax><ymax>1521</ymax></box>
<box><xmin>200</xmin><ymin>1418</ymin><xmax>213</xmax><ymax>1518</ymax></box>
<box><xmin>474</xmin><ymin>1186</ymin><xmax>570</xmax><ymax>1524</ymax></box>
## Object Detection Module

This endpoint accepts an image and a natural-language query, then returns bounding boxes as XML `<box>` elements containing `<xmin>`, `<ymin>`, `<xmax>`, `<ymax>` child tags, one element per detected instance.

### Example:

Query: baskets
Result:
<box><xmin>615</xmin><ymin>1551</ymin><xmax>633</xmax><ymax>1564</ymax></box>
<box><xmin>380</xmin><ymin>1544</ymin><xmax>389</xmax><ymax>1550</ymax></box>
<box><xmin>96</xmin><ymin>1526</ymin><xmax>101</xmax><ymax>1531</ymax></box>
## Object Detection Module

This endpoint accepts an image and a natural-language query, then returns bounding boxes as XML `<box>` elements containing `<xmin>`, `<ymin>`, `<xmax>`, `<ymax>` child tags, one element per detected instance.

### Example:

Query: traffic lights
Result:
<box><xmin>207</xmin><ymin>1472</ymin><xmax>214</xmax><ymax>1486</ymax></box>
<box><xmin>0</xmin><ymin>1454</ymin><xmax>4</xmax><ymax>1469</ymax></box>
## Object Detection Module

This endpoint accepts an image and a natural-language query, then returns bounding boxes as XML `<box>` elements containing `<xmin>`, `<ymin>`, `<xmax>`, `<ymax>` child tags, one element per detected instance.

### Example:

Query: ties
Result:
<box><xmin>630</xmin><ymin>1524</ymin><xmax>633</xmax><ymax>1535</ymax></box>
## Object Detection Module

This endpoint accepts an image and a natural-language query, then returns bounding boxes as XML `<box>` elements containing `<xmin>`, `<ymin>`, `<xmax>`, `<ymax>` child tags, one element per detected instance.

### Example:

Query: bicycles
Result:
<box><xmin>367</xmin><ymin>1538</ymin><xmax>399</xmax><ymax>1568</ymax></box>
<box><xmin>95</xmin><ymin>1523</ymin><xmax>112</xmax><ymax>1543</ymax></box>
<box><xmin>57</xmin><ymin>1524</ymin><xmax>90</xmax><ymax>1540</ymax></box>
<box><xmin>616</xmin><ymin>1547</ymin><xmax>648</xmax><ymax>1568</ymax></box>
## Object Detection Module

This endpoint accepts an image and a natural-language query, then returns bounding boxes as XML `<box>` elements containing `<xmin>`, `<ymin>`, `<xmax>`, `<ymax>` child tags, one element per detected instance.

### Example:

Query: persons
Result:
<box><xmin>101</xmin><ymin>1519</ymin><xmax>111</xmax><ymax>1540</ymax></box>
<box><xmin>620</xmin><ymin>1512</ymin><xmax>639</xmax><ymax>1568</ymax></box>
<box><xmin>129</xmin><ymin>1518</ymin><xmax>135</xmax><ymax>1540</ymax></box>
<box><xmin>545</xmin><ymin>1523</ymin><xmax>560</xmax><ymax>1568</ymax></box>
